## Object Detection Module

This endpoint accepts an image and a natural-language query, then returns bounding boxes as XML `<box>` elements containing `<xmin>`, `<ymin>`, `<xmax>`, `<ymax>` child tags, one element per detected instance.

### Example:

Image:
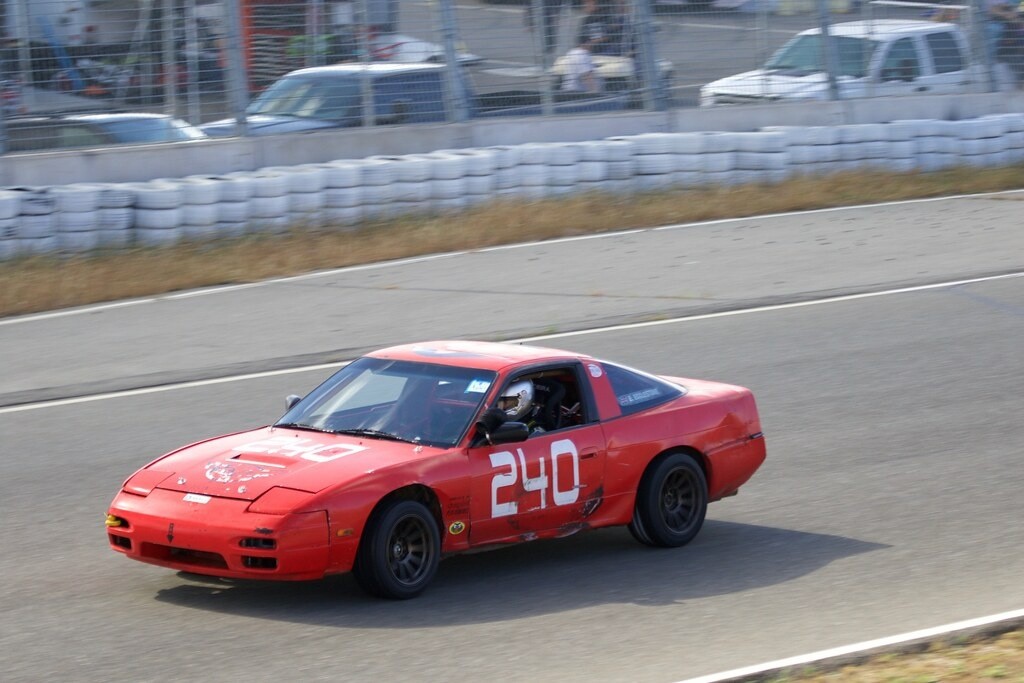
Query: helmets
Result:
<box><xmin>494</xmin><ymin>376</ymin><xmax>535</xmax><ymax>423</ymax></box>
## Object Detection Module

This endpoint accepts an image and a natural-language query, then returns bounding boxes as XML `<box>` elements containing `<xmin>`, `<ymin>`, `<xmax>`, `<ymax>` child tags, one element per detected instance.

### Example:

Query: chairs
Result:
<box><xmin>532</xmin><ymin>377</ymin><xmax>564</xmax><ymax>419</ymax></box>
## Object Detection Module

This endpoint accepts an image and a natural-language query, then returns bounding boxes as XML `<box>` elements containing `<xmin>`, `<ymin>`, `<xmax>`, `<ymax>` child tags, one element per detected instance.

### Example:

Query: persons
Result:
<box><xmin>463</xmin><ymin>378</ymin><xmax>547</xmax><ymax>438</ymax></box>
<box><xmin>980</xmin><ymin>0</ymin><xmax>1024</xmax><ymax>91</ymax></box>
<box><xmin>521</xmin><ymin>0</ymin><xmax>643</xmax><ymax>110</ymax></box>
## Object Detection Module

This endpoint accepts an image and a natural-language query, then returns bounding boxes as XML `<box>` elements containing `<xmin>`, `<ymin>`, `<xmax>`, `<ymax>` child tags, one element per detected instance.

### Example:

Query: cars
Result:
<box><xmin>3</xmin><ymin>110</ymin><xmax>196</xmax><ymax>158</ymax></box>
<box><xmin>100</xmin><ymin>337</ymin><xmax>774</xmax><ymax>598</ymax></box>
<box><xmin>694</xmin><ymin>21</ymin><xmax>1011</xmax><ymax>107</ymax></box>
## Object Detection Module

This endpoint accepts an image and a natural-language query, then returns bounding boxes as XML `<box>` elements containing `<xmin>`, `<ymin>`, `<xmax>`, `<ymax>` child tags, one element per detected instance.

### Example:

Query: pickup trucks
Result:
<box><xmin>201</xmin><ymin>65</ymin><xmax>645</xmax><ymax>139</ymax></box>
